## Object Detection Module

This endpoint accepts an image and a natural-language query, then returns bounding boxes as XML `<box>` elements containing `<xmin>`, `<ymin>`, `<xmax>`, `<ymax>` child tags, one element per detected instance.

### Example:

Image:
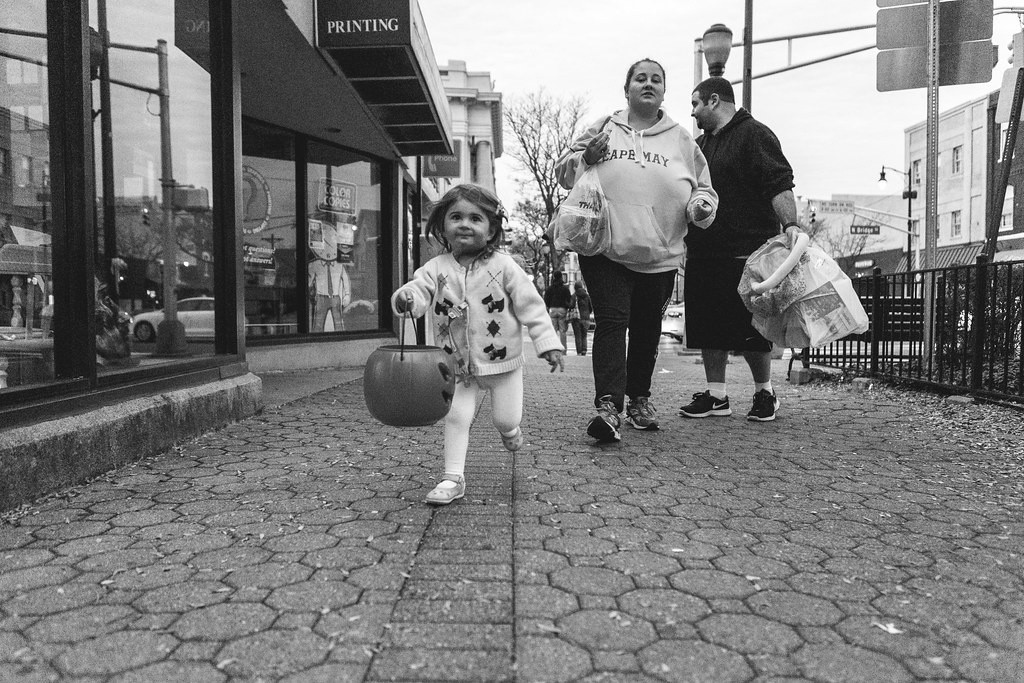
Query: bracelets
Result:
<box><xmin>783</xmin><ymin>222</ymin><xmax>800</xmax><ymax>233</ymax></box>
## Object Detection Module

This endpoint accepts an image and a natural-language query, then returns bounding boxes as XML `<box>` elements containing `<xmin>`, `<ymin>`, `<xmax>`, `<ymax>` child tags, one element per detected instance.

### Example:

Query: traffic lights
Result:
<box><xmin>1008</xmin><ymin>28</ymin><xmax>1024</xmax><ymax>67</ymax></box>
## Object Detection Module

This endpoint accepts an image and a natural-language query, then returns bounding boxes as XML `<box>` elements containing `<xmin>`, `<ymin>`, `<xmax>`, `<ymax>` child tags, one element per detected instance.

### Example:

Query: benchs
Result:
<box><xmin>784</xmin><ymin>297</ymin><xmax>961</xmax><ymax>382</ymax></box>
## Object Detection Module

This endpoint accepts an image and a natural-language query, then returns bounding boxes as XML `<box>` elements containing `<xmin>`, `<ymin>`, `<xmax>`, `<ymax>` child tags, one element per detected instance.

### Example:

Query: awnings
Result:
<box><xmin>894</xmin><ymin>245</ymin><xmax>984</xmax><ymax>279</ymax></box>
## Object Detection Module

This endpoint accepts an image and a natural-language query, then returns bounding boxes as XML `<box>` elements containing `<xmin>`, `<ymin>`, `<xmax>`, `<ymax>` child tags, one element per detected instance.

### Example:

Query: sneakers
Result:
<box><xmin>624</xmin><ymin>396</ymin><xmax>659</xmax><ymax>430</ymax></box>
<box><xmin>587</xmin><ymin>394</ymin><xmax>622</xmax><ymax>442</ymax></box>
<box><xmin>679</xmin><ymin>390</ymin><xmax>732</xmax><ymax>417</ymax></box>
<box><xmin>747</xmin><ymin>386</ymin><xmax>780</xmax><ymax>421</ymax></box>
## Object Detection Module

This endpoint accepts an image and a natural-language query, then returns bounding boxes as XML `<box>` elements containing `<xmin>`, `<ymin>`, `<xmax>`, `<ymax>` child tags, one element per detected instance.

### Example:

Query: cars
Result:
<box><xmin>127</xmin><ymin>296</ymin><xmax>248</xmax><ymax>344</ymax></box>
<box><xmin>659</xmin><ymin>302</ymin><xmax>686</xmax><ymax>342</ymax></box>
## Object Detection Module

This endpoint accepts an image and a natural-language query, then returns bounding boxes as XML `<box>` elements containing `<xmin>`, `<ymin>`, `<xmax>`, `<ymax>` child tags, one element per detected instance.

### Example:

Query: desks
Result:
<box><xmin>244</xmin><ymin>285</ymin><xmax>299</xmax><ymax>335</ymax></box>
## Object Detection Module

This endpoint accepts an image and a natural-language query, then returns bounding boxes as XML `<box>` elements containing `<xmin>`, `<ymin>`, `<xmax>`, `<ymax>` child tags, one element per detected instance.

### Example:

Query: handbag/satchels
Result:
<box><xmin>566</xmin><ymin>296</ymin><xmax>581</xmax><ymax>323</ymax></box>
<box><xmin>553</xmin><ymin>159</ymin><xmax>611</xmax><ymax>256</ymax></box>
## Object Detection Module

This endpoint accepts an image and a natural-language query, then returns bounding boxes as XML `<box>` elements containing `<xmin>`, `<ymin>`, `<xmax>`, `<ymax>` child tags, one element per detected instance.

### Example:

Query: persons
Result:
<box><xmin>544</xmin><ymin>271</ymin><xmax>571</xmax><ymax>355</ymax></box>
<box><xmin>390</xmin><ymin>185</ymin><xmax>565</xmax><ymax>503</ymax></box>
<box><xmin>555</xmin><ymin>58</ymin><xmax>718</xmax><ymax>441</ymax></box>
<box><xmin>533</xmin><ymin>279</ymin><xmax>542</xmax><ymax>296</ymax></box>
<box><xmin>678</xmin><ymin>77</ymin><xmax>804</xmax><ymax>422</ymax></box>
<box><xmin>566</xmin><ymin>281</ymin><xmax>593</xmax><ymax>357</ymax></box>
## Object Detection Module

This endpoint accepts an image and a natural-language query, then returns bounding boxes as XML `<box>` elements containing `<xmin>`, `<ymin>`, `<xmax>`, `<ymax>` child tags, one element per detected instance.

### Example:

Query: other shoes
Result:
<box><xmin>577</xmin><ymin>351</ymin><xmax>586</xmax><ymax>356</ymax></box>
<box><xmin>426</xmin><ymin>475</ymin><xmax>465</xmax><ymax>504</ymax></box>
<box><xmin>499</xmin><ymin>426</ymin><xmax>523</xmax><ymax>450</ymax></box>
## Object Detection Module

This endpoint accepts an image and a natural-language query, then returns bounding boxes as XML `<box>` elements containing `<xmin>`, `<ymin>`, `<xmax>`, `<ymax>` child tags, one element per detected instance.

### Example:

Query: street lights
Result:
<box><xmin>876</xmin><ymin>163</ymin><xmax>912</xmax><ymax>296</ymax></box>
